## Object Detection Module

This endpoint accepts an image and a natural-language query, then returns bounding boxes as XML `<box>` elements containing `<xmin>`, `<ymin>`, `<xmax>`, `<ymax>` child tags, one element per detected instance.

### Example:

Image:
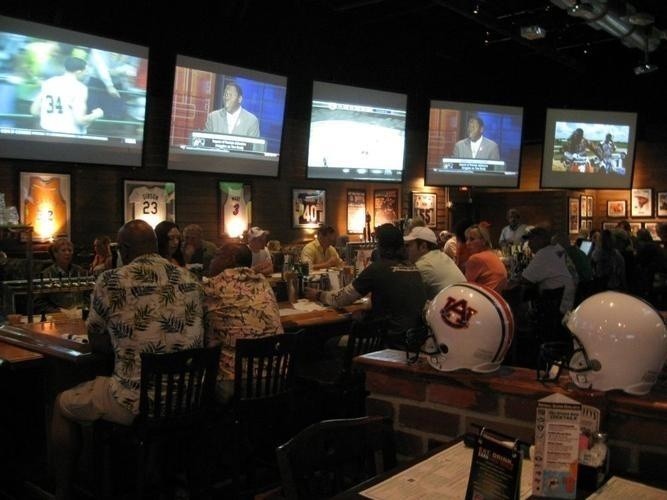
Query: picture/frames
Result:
<box><xmin>409</xmin><ymin>191</ymin><xmax>437</xmax><ymax>230</ymax></box>
<box><xmin>580</xmin><ymin>195</ymin><xmax>593</xmax><ymax>218</ymax></box>
<box><xmin>630</xmin><ymin>188</ymin><xmax>655</xmax><ymax>218</ymax></box>
<box><xmin>346</xmin><ymin>187</ymin><xmax>398</xmax><ymax>234</ymax></box>
<box><xmin>567</xmin><ymin>197</ymin><xmax>580</xmax><ymax>234</ymax></box>
<box><xmin>657</xmin><ymin>191</ymin><xmax>667</xmax><ymax>218</ymax></box>
<box><xmin>580</xmin><ymin>218</ymin><xmax>593</xmax><ymax>230</ymax></box>
<box><xmin>603</xmin><ymin>222</ymin><xmax>662</xmax><ymax>241</ymax></box>
<box><xmin>607</xmin><ymin>200</ymin><xmax>627</xmax><ymax>218</ymax></box>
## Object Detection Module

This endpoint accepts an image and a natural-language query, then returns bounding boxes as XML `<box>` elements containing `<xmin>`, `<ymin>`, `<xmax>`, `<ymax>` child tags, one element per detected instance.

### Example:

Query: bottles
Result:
<box><xmin>590</xmin><ymin>432</ymin><xmax>609</xmax><ymax>480</ymax></box>
<box><xmin>282</xmin><ymin>248</ymin><xmax>310</xmax><ymax>299</ymax></box>
<box><xmin>506</xmin><ymin>240</ymin><xmax>532</xmax><ymax>280</ymax></box>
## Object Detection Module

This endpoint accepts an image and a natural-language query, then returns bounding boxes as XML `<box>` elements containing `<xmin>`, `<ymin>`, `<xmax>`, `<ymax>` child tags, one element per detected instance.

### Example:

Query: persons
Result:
<box><xmin>300</xmin><ymin>211</ymin><xmax>666</xmax><ymax>370</ymax></box>
<box><xmin>1</xmin><ymin>31</ymin><xmax>140</xmax><ymax>134</ymax></box>
<box><xmin>202</xmin><ymin>83</ymin><xmax>260</xmax><ymax>137</ymax></box>
<box><xmin>41</xmin><ymin>219</ymin><xmax>292</xmax><ymax>485</ymax></box>
<box><xmin>454</xmin><ymin>117</ymin><xmax>500</xmax><ymax>160</ymax></box>
<box><xmin>560</xmin><ymin>128</ymin><xmax>616</xmax><ymax>174</ymax></box>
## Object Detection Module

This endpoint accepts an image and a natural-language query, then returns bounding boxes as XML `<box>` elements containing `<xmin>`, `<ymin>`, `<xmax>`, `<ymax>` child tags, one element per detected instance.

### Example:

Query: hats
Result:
<box><xmin>247</xmin><ymin>225</ymin><xmax>271</xmax><ymax>244</ymax></box>
<box><xmin>402</xmin><ymin>226</ymin><xmax>439</xmax><ymax>246</ymax></box>
<box><xmin>520</xmin><ymin>227</ymin><xmax>550</xmax><ymax>242</ymax></box>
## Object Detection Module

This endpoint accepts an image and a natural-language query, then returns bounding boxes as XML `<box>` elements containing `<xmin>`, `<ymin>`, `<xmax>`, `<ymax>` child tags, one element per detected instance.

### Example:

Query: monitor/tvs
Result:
<box><xmin>579</xmin><ymin>239</ymin><xmax>593</xmax><ymax>256</ymax></box>
<box><xmin>538</xmin><ymin>104</ymin><xmax>642</xmax><ymax>190</ymax></box>
<box><xmin>1</xmin><ymin>10</ymin><xmax>155</xmax><ymax>171</ymax></box>
<box><xmin>424</xmin><ymin>93</ymin><xmax>527</xmax><ymax>189</ymax></box>
<box><xmin>305</xmin><ymin>74</ymin><xmax>412</xmax><ymax>184</ymax></box>
<box><xmin>164</xmin><ymin>51</ymin><xmax>290</xmax><ymax>181</ymax></box>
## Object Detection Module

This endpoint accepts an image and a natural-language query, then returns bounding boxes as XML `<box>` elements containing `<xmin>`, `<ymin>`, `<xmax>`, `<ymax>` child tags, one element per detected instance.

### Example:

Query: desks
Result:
<box><xmin>332</xmin><ymin>433</ymin><xmax>667</xmax><ymax>500</ymax></box>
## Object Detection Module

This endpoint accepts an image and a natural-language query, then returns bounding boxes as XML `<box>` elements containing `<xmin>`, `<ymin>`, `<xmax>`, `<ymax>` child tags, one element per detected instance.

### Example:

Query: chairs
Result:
<box><xmin>234</xmin><ymin>327</ymin><xmax>303</xmax><ymax>404</ymax></box>
<box><xmin>135</xmin><ymin>339</ymin><xmax>225</xmax><ymax>430</ymax></box>
<box><xmin>278</xmin><ymin>414</ymin><xmax>393</xmax><ymax>500</ymax></box>
<box><xmin>348</xmin><ymin>316</ymin><xmax>391</xmax><ymax>358</ymax></box>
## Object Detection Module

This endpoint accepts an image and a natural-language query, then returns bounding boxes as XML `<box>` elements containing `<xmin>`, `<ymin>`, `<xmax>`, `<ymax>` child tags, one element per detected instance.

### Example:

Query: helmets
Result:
<box><xmin>535</xmin><ymin>289</ymin><xmax>667</xmax><ymax>398</ymax></box>
<box><xmin>403</xmin><ymin>280</ymin><xmax>518</xmax><ymax>376</ymax></box>
